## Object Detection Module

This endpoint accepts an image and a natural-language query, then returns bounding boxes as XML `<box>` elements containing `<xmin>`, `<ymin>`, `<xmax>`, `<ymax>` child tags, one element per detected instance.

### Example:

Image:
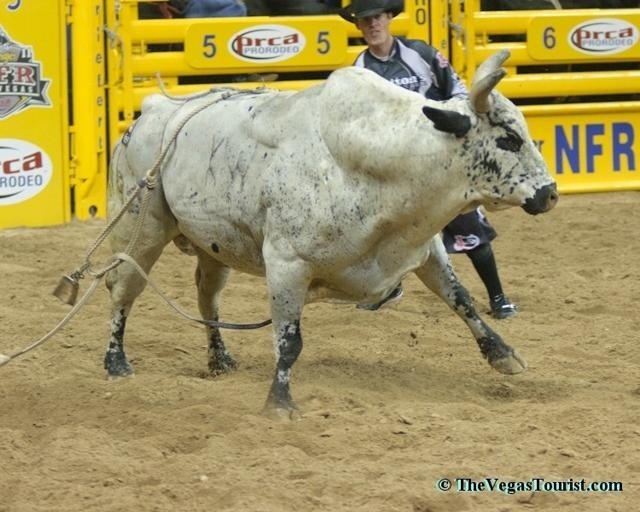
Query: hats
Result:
<box><xmin>336</xmin><ymin>0</ymin><xmax>405</xmax><ymax>23</ymax></box>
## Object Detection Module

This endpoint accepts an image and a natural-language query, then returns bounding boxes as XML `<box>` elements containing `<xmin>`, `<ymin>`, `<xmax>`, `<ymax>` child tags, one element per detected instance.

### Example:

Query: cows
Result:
<box><xmin>102</xmin><ymin>47</ymin><xmax>560</xmax><ymax>428</ymax></box>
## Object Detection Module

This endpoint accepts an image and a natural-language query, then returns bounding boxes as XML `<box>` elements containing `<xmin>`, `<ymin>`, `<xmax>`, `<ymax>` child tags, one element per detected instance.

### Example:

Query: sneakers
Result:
<box><xmin>488</xmin><ymin>296</ymin><xmax>520</xmax><ymax>320</ymax></box>
<box><xmin>355</xmin><ymin>280</ymin><xmax>406</xmax><ymax>311</ymax></box>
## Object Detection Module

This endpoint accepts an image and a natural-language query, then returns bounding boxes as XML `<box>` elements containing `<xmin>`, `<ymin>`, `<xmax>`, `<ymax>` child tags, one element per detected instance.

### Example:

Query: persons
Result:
<box><xmin>336</xmin><ymin>0</ymin><xmax>521</xmax><ymax>321</ymax></box>
<box><xmin>135</xmin><ymin>0</ymin><xmax>349</xmax><ymax>84</ymax></box>
